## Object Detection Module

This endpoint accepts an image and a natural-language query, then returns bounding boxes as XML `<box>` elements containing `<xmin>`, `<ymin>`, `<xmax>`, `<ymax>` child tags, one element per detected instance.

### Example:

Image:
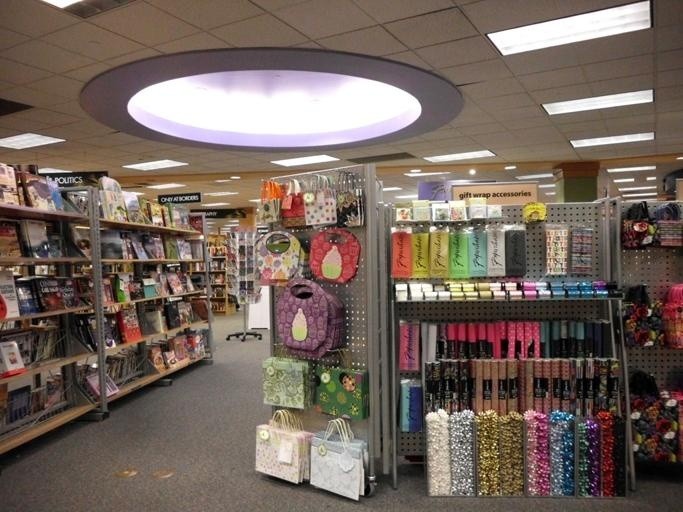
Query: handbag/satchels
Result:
<box><xmin>623</xmin><ymin>371</ymin><xmax>682</xmax><ymax>469</ymax></box>
<box><xmin>283</xmin><ymin>287</ymin><xmax>347</xmax><ymax>360</ymax></box>
<box><xmin>254</xmin><ymin>409</ymin><xmax>313</xmax><ymax>485</ymax></box>
<box><xmin>253</xmin><ymin>230</ymin><xmax>301</xmax><ymax>282</ymax></box>
<box><xmin>313</xmin><ymin>349</ymin><xmax>370</xmax><ymax>421</ymax></box>
<box><xmin>257</xmin><ymin>179</ymin><xmax>281</xmax><ymax>224</ymax></box>
<box><xmin>303</xmin><ymin>174</ymin><xmax>337</xmax><ymax>226</ymax></box>
<box><xmin>622</xmin><ymin>201</ymin><xmax>659</xmax><ymax>250</ymax></box>
<box><xmin>308</xmin><ymin>418</ymin><xmax>371</xmax><ymax>502</ymax></box>
<box><xmin>277</xmin><ymin>279</ymin><xmax>329</xmax><ymax>352</ymax></box>
<box><xmin>616</xmin><ymin>285</ymin><xmax>667</xmax><ymax>347</ymax></box>
<box><xmin>654</xmin><ymin>200</ymin><xmax>683</xmax><ymax>248</ymax></box>
<box><xmin>665</xmin><ymin>285</ymin><xmax>683</xmax><ymax>348</ymax></box>
<box><xmin>281</xmin><ymin>177</ymin><xmax>304</xmax><ymax>218</ymax></box>
<box><xmin>336</xmin><ymin>171</ymin><xmax>366</xmax><ymax>226</ymax></box>
<box><xmin>261</xmin><ymin>344</ymin><xmax>315</xmax><ymax>410</ymax></box>
<box><xmin>308</xmin><ymin>227</ymin><xmax>361</xmax><ymax>286</ymax></box>
<box><xmin>253</xmin><ymin>241</ymin><xmax>306</xmax><ymax>288</ymax></box>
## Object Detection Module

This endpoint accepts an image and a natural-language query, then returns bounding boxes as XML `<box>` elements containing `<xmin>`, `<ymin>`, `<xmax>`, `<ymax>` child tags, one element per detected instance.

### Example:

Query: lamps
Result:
<box><xmin>569</xmin><ymin>132</ymin><xmax>655</xmax><ymax>148</ymax></box>
<box><xmin>270</xmin><ymin>154</ymin><xmax>341</xmax><ymax>167</ymax></box>
<box><xmin>422</xmin><ymin>149</ymin><xmax>496</xmax><ymax>163</ymax></box>
<box><xmin>121</xmin><ymin>159</ymin><xmax>190</xmax><ymax>171</ymax></box>
<box><xmin>0</xmin><ymin>132</ymin><xmax>67</xmax><ymax>149</ymax></box>
<box><xmin>79</xmin><ymin>47</ymin><xmax>463</xmax><ymax>152</ymax></box>
<box><xmin>540</xmin><ymin>88</ymin><xmax>654</xmax><ymax>115</ymax></box>
<box><xmin>484</xmin><ymin>0</ymin><xmax>653</xmax><ymax>57</ymax></box>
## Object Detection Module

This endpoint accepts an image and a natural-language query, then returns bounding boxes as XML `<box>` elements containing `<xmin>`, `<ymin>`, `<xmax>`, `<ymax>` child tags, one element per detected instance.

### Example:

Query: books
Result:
<box><xmin>75</xmin><ymin>315</ymin><xmax>121</xmax><ymax>350</ymax></box>
<box><xmin>162</xmin><ymin>207</ymin><xmax>172</xmax><ymax>227</ymax></box>
<box><xmin>8</xmin><ymin>385</ymin><xmax>30</xmax><ymax>424</ymax></box>
<box><xmin>21</xmin><ymin>172</ymin><xmax>56</xmax><ymax>210</ymax></box>
<box><xmin>99</xmin><ymin>230</ymin><xmax>124</xmax><ymax>259</ymax></box>
<box><xmin>163</xmin><ymin>351</ymin><xmax>179</xmax><ymax>368</ymax></box>
<box><xmin>0</xmin><ymin>384</ymin><xmax>8</xmax><ymax>432</ymax></box>
<box><xmin>138</xmin><ymin>311</ymin><xmax>163</xmax><ymax>335</ymax></box>
<box><xmin>17</xmin><ymin>275</ymin><xmax>114</xmax><ymax>313</ymax></box>
<box><xmin>165</xmin><ymin>303</ymin><xmax>181</xmax><ymax>329</ymax></box>
<box><xmin>168</xmin><ymin>336</ymin><xmax>187</xmax><ymax>362</ymax></box>
<box><xmin>0</xmin><ymin>222</ymin><xmax>23</xmax><ymax>257</ymax></box>
<box><xmin>116</xmin><ymin>310</ymin><xmax>142</xmax><ymax>344</ymax></box>
<box><xmin>0</xmin><ymin>327</ymin><xmax>57</xmax><ymax>377</ymax></box>
<box><xmin>30</xmin><ymin>388</ymin><xmax>48</xmax><ymax>420</ymax></box>
<box><xmin>153</xmin><ymin>238</ymin><xmax>165</xmax><ymax>259</ymax></box>
<box><xmin>21</xmin><ymin>220</ymin><xmax>51</xmax><ymax>258</ymax></box>
<box><xmin>162</xmin><ymin>233</ymin><xmax>180</xmax><ymax>259</ymax></box>
<box><xmin>16</xmin><ymin>171</ymin><xmax>28</xmax><ymax>206</ymax></box>
<box><xmin>178</xmin><ymin>240</ymin><xmax>192</xmax><ymax>259</ymax></box>
<box><xmin>0</xmin><ymin>271</ymin><xmax>20</xmax><ymax>319</ymax></box>
<box><xmin>0</xmin><ymin>162</ymin><xmax>19</xmax><ymax>205</ymax></box>
<box><xmin>48</xmin><ymin>181</ymin><xmax>64</xmax><ymax>211</ymax></box>
<box><xmin>147</xmin><ymin>203</ymin><xmax>164</xmax><ymax>227</ymax></box>
<box><xmin>149</xmin><ymin>347</ymin><xmax>165</xmax><ymax>371</ymax></box>
<box><xmin>85</xmin><ymin>372</ymin><xmax>118</xmax><ymax>401</ymax></box>
<box><xmin>121</xmin><ymin>191</ymin><xmax>144</xmax><ymax>224</ymax></box>
<box><xmin>138</xmin><ymin>196</ymin><xmax>151</xmax><ymax>224</ymax></box>
<box><xmin>46</xmin><ymin>374</ymin><xmax>64</xmax><ymax>413</ymax></box>
<box><xmin>100</xmin><ymin>191</ymin><xmax>127</xmax><ymax>221</ymax></box>
<box><xmin>167</xmin><ymin>274</ymin><xmax>186</xmax><ymax>294</ymax></box>
<box><xmin>133</xmin><ymin>240</ymin><xmax>148</xmax><ymax>259</ymax></box>
<box><xmin>187</xmin><ymin>333</ymin><xmax>205</xmax><ymax>360</ymax></box>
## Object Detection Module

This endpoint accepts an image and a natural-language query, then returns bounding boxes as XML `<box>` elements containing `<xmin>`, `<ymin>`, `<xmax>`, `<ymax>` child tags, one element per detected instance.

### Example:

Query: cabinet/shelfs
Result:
<box><xmin>0</xmin><ymin>186</ymin><xmax>105</xmax><ymax>454</ymax></box>
<box><xmin>206</xmin><ymin>234</ymin><xmax>236</xmax><ymax>315</ymax></box>
<box><xmin>88</xmin><ymin>188</ymin><xmax>213</xmax><ymax>418</ymax></box>
<box><xmin>609</xmin><ymin>196</ymin><xmax>683</xmax><ymax>492</ymax></box>
<box><xmin>269</xmin><ymin>162</ymin><xmax>629</xmax><ymax>491</ymax></box>
<box><xmin>239</xmin><ymin>236</ymin><xmax>254</xmax><ymax>306</ymax></box>
